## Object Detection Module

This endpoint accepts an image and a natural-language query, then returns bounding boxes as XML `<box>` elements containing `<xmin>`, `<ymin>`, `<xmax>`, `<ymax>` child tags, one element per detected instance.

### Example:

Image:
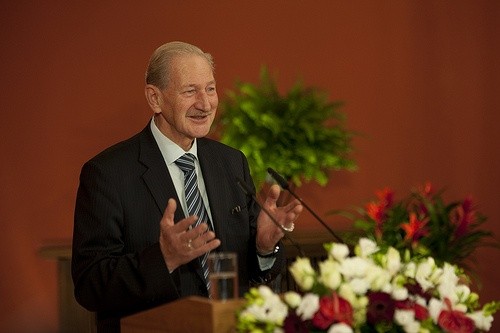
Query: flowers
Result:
<box><xmin>234</xmin><ymin>238</ymin><xmax>499</xmax><ymax>332</ymax></box>
<box><xmin>324</xmin><ymin>176</ymin><xmax>497</xmax><ymax>276</ymax></box>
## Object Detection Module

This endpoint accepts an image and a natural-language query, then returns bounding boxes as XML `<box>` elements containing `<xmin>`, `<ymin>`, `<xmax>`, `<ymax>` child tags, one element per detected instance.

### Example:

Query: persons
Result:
<box><xmin>71</xmin><ymin>41</ymin><xmax>302</xmax><ymax>333</ymax></box>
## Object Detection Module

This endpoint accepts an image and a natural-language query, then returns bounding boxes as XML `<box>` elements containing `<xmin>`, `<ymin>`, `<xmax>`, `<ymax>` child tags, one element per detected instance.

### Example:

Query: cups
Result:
<box><xmin>207</xmin><ymin>253</ymin><xmax>239</xmax><ymax>300</ymax></box>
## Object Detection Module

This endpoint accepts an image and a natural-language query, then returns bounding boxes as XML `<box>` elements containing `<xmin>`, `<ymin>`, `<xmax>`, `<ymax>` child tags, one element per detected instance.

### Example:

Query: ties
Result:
<box><xmin>174</xmin><ymin>153</ymin><xmax>223</xmax><ymax>299</ymax></box>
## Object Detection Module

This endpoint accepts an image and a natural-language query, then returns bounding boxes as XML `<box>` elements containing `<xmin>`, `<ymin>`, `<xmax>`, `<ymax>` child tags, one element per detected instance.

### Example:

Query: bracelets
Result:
<box><xmin>258</xmin><ymin>241</ymin><xmax>280</xmax><ymax>258</ymax></box>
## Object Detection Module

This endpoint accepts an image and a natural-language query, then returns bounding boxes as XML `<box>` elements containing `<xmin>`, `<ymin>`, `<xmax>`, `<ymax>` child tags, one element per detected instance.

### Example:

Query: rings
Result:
<box><xmin>282</xmin><ymin>221</ymin><xmax>294</xmax><ymax>231</ymax></box>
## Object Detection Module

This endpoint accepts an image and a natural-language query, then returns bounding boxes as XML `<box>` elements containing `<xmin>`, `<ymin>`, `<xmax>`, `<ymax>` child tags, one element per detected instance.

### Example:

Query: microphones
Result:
<box><xmin>267</xmin><ymin>168</ymin><xmax>345</xmax><ymax>244</ymax></box>
<box><xmin>235</xmin><ymin>175</ymin><xmax>306</xmax><ymax>257</ymax></box>
